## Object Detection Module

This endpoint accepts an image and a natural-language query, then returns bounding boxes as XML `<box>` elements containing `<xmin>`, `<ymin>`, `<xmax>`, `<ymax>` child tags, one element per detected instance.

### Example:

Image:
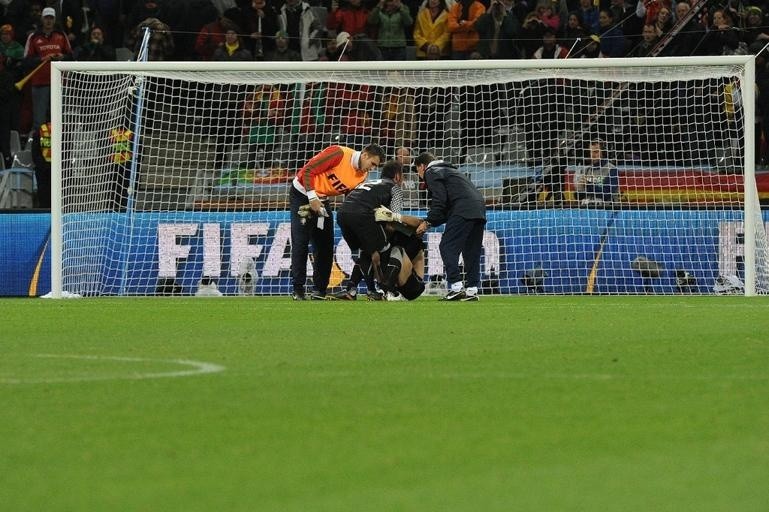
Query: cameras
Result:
<box><xmin>531</xmin><ymin>19</ymin><xmax>537</xmax><ymax>25</ymax></box>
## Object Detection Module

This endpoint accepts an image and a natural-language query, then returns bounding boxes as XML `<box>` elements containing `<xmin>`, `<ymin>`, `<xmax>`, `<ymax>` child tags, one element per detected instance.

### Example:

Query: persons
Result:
<box><xmin>337</xmin><ymin>159</ymin><xmax>405</xmax><ymax>302</ymax></box>
<box><xmin>360</xmin><ymin>204</ymin><xmax>428</xmax><ymax>301</ymax></box>
<box><xmin>1</xmin><ymin>0</ymin><xmax>769</xmax><ymax>210</ymax></box>
<box><xmin>412</xmin><ymin>149</ymin><xmax>489</xmax><ymax>303</ymax></box>
<box><xmin>287</xmin><ymin>144</ymin><xmax>389</xmax><ymax>302</ymax></box>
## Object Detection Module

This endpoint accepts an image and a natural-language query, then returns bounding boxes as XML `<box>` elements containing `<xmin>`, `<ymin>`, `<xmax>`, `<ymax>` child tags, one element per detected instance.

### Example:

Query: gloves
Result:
<box><xmin>373</xmin><ymin>205</ymin><xmax>401</xmax><ymax>221</ymax></box>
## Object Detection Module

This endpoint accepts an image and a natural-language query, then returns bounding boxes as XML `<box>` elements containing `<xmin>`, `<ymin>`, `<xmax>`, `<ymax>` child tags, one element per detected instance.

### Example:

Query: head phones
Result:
<box><xmin>594</xmin><ymin>137</ymin><xmax>610</xmax><ymax>158</ymax></box>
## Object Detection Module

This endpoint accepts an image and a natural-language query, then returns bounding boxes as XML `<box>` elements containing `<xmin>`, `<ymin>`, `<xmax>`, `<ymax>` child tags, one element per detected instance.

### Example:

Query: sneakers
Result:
<box><xmin>292</xmin><ymin>289</ymin><xmax>409</xmax><ymax>301</ymax></box>
<box><xmin>438</xmin><ymin>289</ymin><xmax>479</xmax><ymax>301</ymax></box>
